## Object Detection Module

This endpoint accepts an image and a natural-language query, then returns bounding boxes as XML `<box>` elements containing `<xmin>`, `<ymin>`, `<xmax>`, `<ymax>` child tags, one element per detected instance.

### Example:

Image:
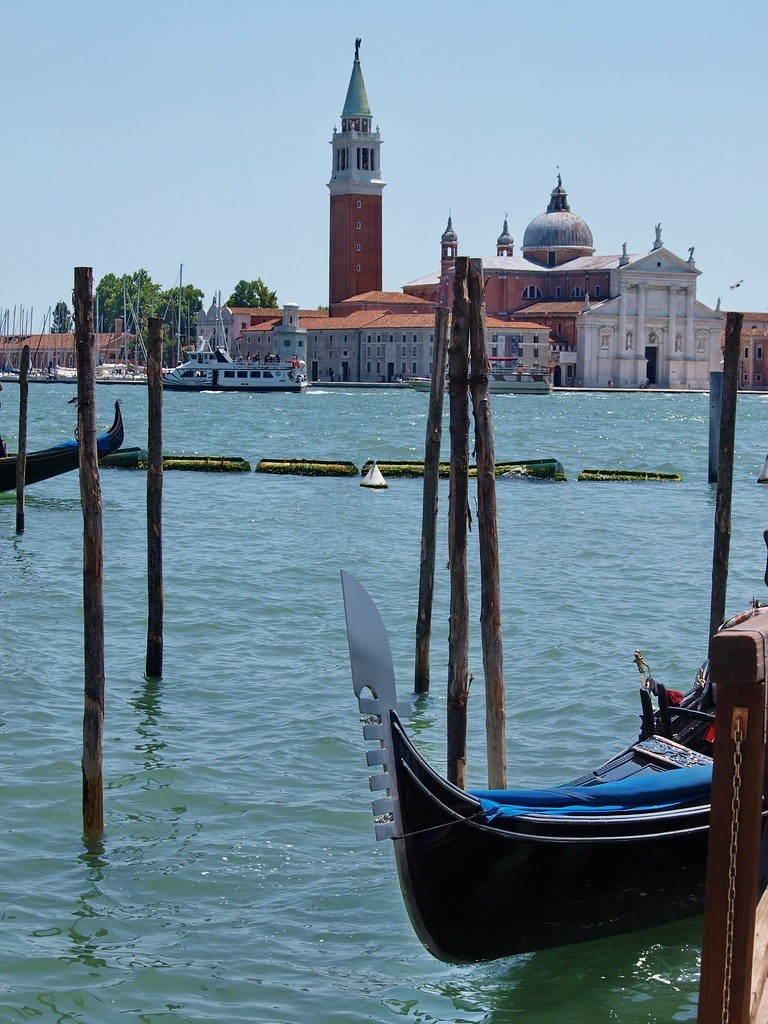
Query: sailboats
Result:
<box><xmin>0</xmin><ymin>264</ymin><xmax>230</xmax><ymax>383</ymax></box>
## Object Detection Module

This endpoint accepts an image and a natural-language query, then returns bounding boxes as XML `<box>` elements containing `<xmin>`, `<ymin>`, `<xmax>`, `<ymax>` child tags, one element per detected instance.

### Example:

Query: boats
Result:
<box><xmin>160</xmin><ymin>336</ymin><xmax>309</xmax><ymax>391</ymax></box>
<box><xmin>0</xmin><ymin>398</ymin><xmax>125</xmax><ymax>494</ymax></box>
<box><xmin>339</xmin><ymin>566</ymin><xmax>768</xmax><ymax>969</ymax></box>
<box><xmin>407</xmin><ymin>356</ymin><xmax>554</xmax><ymax>394</ymax></box>
